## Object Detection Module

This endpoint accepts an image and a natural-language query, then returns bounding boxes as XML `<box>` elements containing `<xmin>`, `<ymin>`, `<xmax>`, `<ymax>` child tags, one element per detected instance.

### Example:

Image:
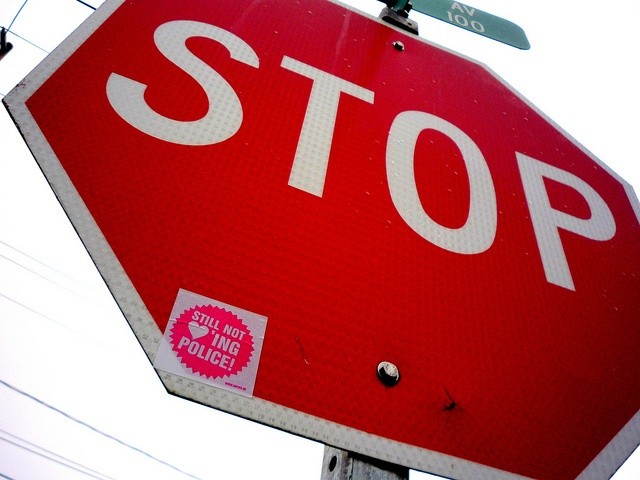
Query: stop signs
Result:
<box><xmin>1</xmin><ymin>0</ymin><xmax>640</xmax><ymax>475</ymax></box>
